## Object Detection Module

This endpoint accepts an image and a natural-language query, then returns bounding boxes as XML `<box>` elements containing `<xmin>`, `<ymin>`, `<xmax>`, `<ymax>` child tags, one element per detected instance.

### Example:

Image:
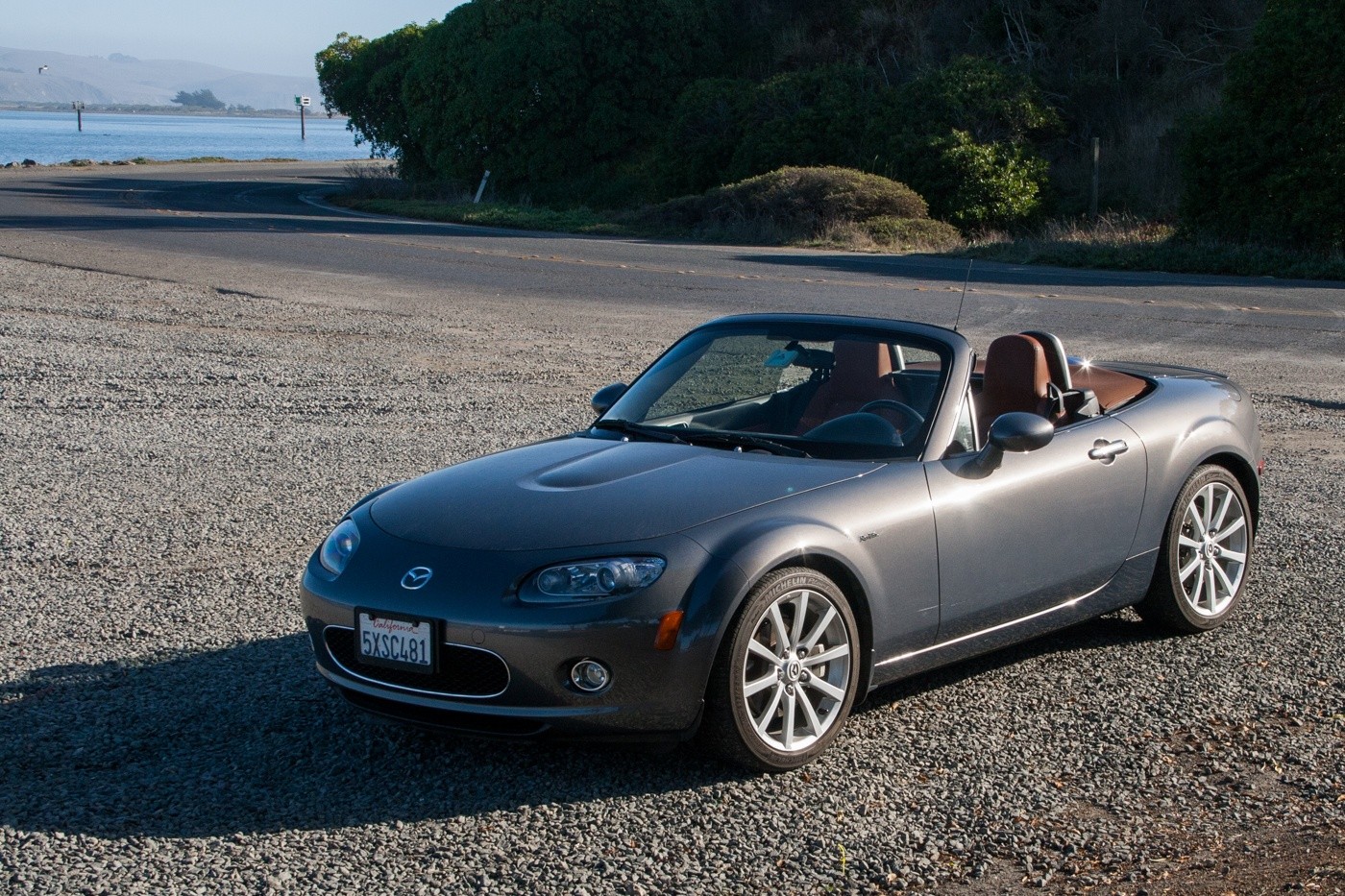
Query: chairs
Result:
<box><xmin>962</xmin><ymin>333</ymin><xmax>1066</xmax><ymax>450</ymax></box>
<box><xmin>796</xmin><ymin>336</ymin><xmax>897</xmax><ymax>434</ymax></box>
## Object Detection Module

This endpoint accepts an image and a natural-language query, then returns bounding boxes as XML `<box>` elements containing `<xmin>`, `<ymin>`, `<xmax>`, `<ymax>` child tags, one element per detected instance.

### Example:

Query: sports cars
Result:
<box><xmin>299</xmin><ymin>313</ymin><xmax>1264</xmax><ymax>774</ymax></box>
